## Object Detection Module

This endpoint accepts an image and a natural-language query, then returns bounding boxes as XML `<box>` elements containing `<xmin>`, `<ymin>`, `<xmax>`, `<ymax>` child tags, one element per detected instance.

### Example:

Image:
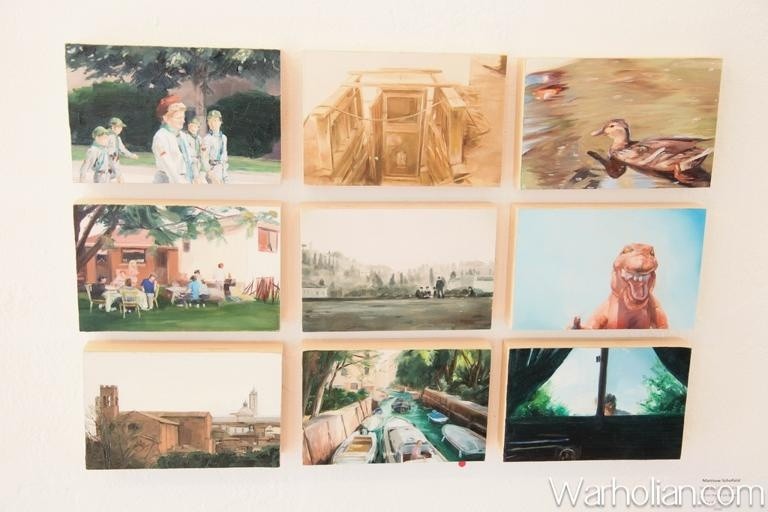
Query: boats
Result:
<box><xmin>440</xmin><ymin>423</ymin><xmax>486</xmax><ymax>460</ymax></box>
<box><xmin>360</xmin><ymin>407</ymin><xmax>386</xmax><ymax>432</ymax></box>
<box><xmin>330</xmin><ymin>427</ymin><xmax>379</xmax><ymax>464</ymax></box>
<box><xmin>391</xmin><ymin>397</ymin><xmax>412</xmax><ymax>414</ymax></box>
<box><xmin>426</xmin><ymin>409</ymin><xmax>450</xmax><ymax>426</ymax></box>
<box><xmin>381</xmin><ymin>416</ymin><xmax>449</xmax><ymax>463</ymax></box>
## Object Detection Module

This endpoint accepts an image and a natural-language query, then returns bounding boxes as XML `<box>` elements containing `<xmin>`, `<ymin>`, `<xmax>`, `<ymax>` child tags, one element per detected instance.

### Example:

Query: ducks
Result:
<box><xmin>591</xmin><ymin>119</ymin><xmax>715</xmax><ymax>185</ymax></box>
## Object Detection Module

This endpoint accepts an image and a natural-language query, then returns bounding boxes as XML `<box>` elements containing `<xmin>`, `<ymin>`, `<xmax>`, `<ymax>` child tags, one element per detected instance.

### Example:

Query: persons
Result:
<box><xmin>91</xmin><ymin>259</ymin><xmax>227</xmax><ymax>314</ymax></box>
<box><xmin>199</xmin><ymin>110</ymin><xmax>231</xmax><ymax>185</ymax></box>
<box><xmin>80</xmin><ymin>126</ymin><xmax>113</xmax><ymax>182</ymax></box>
<box><xmin>412</xmin><ymin>270</ymin><xmax>476</xmax><ymax>299</ymax></box>
<box><xmin>603</xmin><ymin>393</ymin><xmax>631</xmax><ymax>416</ymax></box>
<box><xmin>106</xmin><ymin>116</ymin><xmax>139</xmax><ymax>181</ymax></box>
<box><xmin>182</xmin><ymin>116</ymin><xmax>203</xmax><ymax>184</ymax></box>
<box><xmin>151</xmin><ymin>94</ymin><xmax>196</xmax><ymax>184</ymax></box>
